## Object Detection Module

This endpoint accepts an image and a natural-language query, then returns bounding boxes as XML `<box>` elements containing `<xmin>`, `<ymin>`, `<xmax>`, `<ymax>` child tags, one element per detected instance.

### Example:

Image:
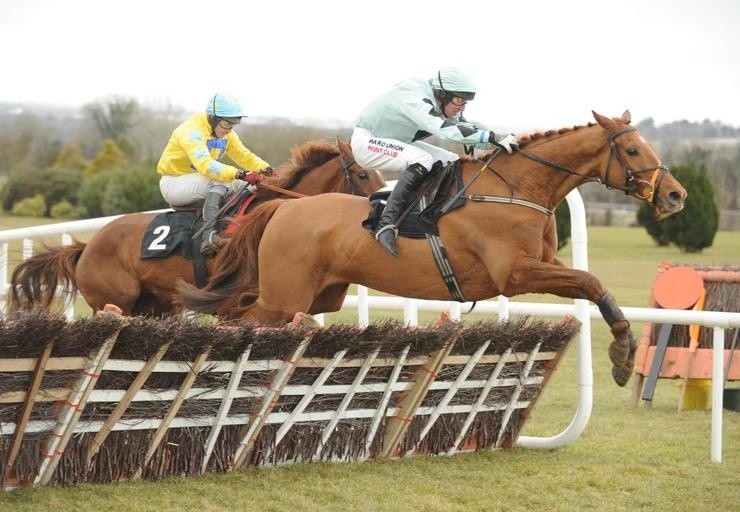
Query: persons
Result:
<box><xmin>350</xmin><ymin>69</ymin><xmax>519</xmax><ymax>256</ymax></box>
<box><xmin>156</xmin><ymin>94</ymin><xmax>280</xmax><ymax>257</ymax></box>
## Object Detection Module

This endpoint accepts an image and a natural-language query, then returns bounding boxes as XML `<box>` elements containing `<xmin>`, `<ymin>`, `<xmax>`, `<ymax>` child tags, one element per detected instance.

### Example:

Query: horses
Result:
<box><xmin>172</xmin><ymin>110</ymin><xmax>687</xmax><ymax>387</ymax></box>
<box><xmin>0</xmin><ymin>135</ymin><xmax>387</xmax><ymax>320</ymax></box>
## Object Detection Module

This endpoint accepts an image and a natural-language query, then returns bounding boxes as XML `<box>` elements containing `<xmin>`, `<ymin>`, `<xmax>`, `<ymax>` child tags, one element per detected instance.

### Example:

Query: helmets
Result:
<box><xmin>431</xmin><ymin>65</ymin><xmax>477</xmax><ymax>101</ymax></box>
<box><xmin>206</xmin><ymin>94</ymin><xmax>250</xmax><ymax>125</ymax></box>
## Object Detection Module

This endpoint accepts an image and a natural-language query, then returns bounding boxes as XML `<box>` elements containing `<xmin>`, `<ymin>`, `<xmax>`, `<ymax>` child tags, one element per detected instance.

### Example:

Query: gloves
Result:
<box><xmin>488</xmin><ymin>129</ymin><xmax>520</xmax><ymax>155</ymax></box>
<box><xmin>263</xmin><ymin>166</ymin><xmax>281</xmax><ymax>180</ymax></box>
<box><xmin>235</xmin><ymin>168</ymin><xmax>263</xmax><ymax>186</ymax></box>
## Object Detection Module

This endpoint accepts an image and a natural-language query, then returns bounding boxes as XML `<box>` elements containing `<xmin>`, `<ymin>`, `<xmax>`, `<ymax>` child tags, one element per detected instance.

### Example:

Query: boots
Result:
<box><xmin>199</xmin><ymin>191</ymin><xmax>232</xmax><ymax>255</ymax></box>
<box><xmin>368</xmin><ymin>161</ymin><xmax>429</xmax><ymax>257</ymax></box>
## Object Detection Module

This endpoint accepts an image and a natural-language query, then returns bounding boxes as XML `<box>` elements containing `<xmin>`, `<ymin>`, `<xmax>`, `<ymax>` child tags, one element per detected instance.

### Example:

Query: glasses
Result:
<box><xmin>449</xmin><ymin>95</ymin><xmax>468</xmax><ymax>105</ymax></box>
<box><xmin>216</xmin><ymin>118</ymin><xmax>235</xmax><ymax>128</ymax></box>
